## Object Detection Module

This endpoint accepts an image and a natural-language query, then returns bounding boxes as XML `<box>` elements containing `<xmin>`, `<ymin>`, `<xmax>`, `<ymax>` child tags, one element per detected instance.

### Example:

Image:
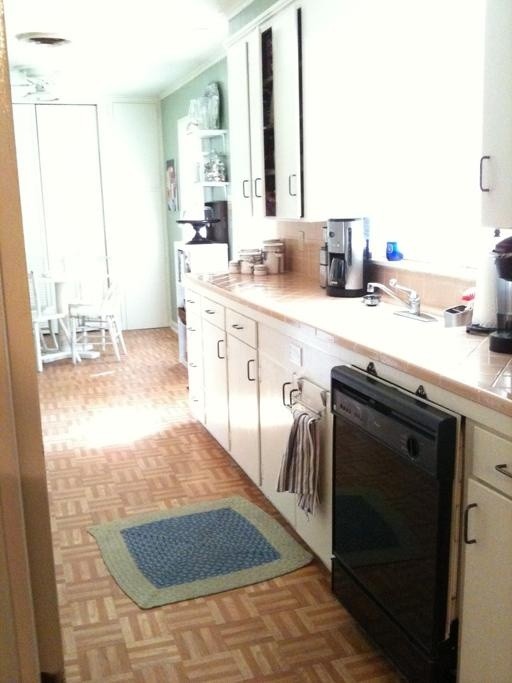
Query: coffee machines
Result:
<box><xmin>327</xmin><ymin>218</ymin><xmax>369</xmax><ymax>297</ymax></box>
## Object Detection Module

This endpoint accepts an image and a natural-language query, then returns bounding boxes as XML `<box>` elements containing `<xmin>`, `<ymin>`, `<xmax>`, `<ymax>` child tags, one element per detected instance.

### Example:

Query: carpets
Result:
<box><xmin>86</xmin><ymin>495</ymin><xmax>314</xmax><ymax>609</ymax></box>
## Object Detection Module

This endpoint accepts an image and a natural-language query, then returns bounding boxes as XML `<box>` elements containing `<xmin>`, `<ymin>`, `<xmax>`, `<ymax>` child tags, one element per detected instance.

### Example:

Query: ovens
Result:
<box><xmin>332</xmin><ymin>365</ymin><xmax>465</xmax><ymax>683</ymax></box>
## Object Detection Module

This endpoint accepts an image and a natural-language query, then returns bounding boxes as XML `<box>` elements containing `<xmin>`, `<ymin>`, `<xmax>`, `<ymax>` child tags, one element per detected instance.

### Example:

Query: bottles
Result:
<box><xmin>229</xmin><ymin>240</ymin><xmax>284</xmax><ymax>275</ymax></box>
<box><xmin>201</xmin><ymin>150</ymin><xmax>228</xmax><ymax>182</ymax></box>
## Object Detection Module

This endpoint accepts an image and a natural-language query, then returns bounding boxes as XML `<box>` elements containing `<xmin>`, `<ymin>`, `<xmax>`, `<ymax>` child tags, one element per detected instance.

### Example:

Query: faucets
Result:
<box><xmin>367</xmin><ymin>278</ymin><xmax>421</xmax><ymax>316</ymax></box>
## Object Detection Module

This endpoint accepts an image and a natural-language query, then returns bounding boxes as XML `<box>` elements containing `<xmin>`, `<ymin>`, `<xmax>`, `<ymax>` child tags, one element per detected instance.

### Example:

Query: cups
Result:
<box><xmin>386</xmin><ymin>241</ymin><xmax>398</xmax><ymax>261</ymax></box>
<box><xmin>189</xmin><ymin>83</ymin><xmax>220</xmax><ymax>129</ymax></box>
<box><xmin>363</xmin><ymin>295</ymin><xmax>380</xmax><ymax>306</ymax></box>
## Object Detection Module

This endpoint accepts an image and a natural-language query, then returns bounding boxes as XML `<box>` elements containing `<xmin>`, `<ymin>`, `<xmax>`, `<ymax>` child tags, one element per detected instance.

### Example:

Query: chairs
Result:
<box><xmin>27</xmin><ymin>264</ymin><xmax>129</xmax><ymax>372</ymax></box>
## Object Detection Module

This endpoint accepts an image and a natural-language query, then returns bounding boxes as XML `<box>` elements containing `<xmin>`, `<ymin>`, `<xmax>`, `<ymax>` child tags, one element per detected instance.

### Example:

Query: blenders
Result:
<box><xmin>490</xmin><ymin>236</ymin><xmax>512</xmax><ymax>354</ymax></box>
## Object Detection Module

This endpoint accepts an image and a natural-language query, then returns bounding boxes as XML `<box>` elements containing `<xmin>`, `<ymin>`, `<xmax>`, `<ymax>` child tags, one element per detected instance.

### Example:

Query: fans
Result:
<box><xmin>6</xmin><ymin>31</ymin><xmax>88</xmax><ymax>110</ymax></box>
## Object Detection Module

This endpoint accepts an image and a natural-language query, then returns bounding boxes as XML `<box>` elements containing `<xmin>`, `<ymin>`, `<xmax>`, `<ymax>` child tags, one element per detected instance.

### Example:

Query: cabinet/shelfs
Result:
<box><xmin>480</xmin><ymin>0</ymin><xmax>512</xmax><ymax>228</ymax></box>
<box><xmin>189</xmin><ymin>129</ymin><xmax>230</xmax><ymax>187</ymax></box>
<box><xmin>226</xmin><ymin>0</ymin><xmax>306</xmax><ymax>219</ymax></box>
<box><xmin>173</xmin><ymin>240</ymin><xmax>229</xmax><ymax>369</ymax></box>
